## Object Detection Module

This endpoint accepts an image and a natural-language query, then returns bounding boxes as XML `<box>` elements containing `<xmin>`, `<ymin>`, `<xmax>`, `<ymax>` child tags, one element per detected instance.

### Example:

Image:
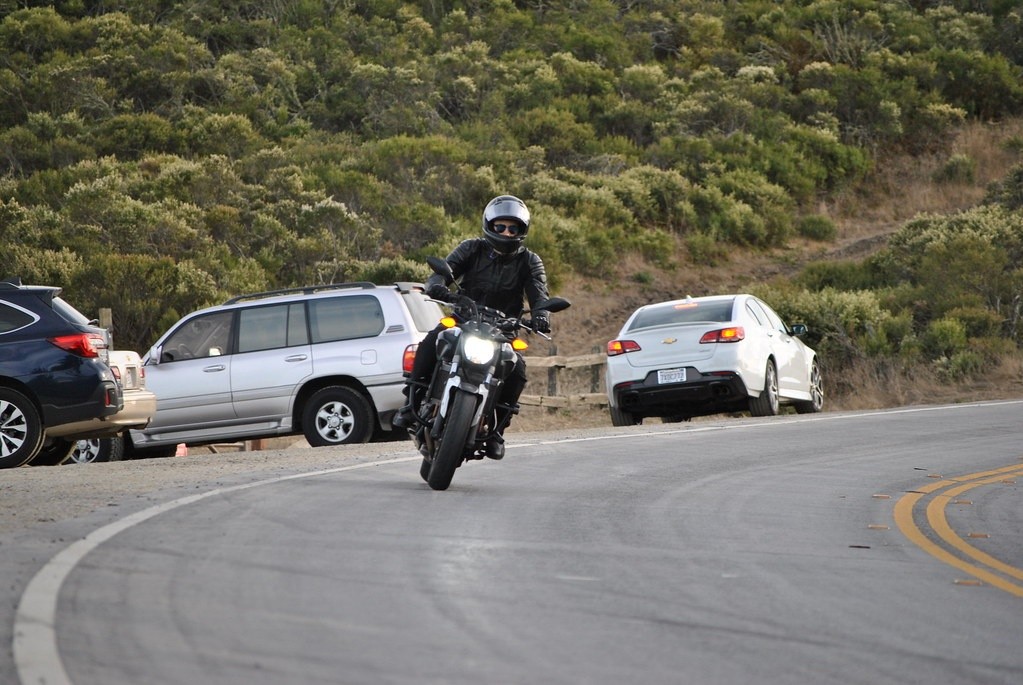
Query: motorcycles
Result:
<box><xmin>398</xmin><ymin>256</ymin><xmax>571</xmax><ymax>491</ymax></box>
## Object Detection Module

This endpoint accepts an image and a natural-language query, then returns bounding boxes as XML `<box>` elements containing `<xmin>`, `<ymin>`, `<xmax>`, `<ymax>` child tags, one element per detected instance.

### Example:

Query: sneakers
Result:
<box><xmin>486</xmin><ymin>432</ymin><xmax>506</xmax><ymax>460</ymax></box>
<box><xmin>392</xmin><ymin>398</ymin><xmax>421</xmax><ymax>428</ymax></box>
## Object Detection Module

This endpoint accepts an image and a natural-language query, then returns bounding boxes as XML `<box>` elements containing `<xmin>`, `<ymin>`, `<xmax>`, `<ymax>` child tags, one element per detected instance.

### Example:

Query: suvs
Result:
<box><xmin>109</xmin><ymin>280</ymin><xmax>445</xmax><ymax>446</ymax></box>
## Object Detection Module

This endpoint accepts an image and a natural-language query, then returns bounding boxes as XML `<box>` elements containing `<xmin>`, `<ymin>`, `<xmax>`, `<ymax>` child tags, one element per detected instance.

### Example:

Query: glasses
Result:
<box><xmin>495</xmin><ymin>224</ymin><xmax>519</xmax><ymax>235</ymax></box>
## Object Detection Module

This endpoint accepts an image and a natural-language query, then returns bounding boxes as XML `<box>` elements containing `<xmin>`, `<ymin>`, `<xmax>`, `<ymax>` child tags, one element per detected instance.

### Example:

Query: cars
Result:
<box><xmin>606</xmin><ymin>293</ymin><xmax>826</xmax><ymax>426</ymax></box>
<box><xmin>59</xmin><ymin>351</ymin><xmax>155</xmax><ymax>463</ymax></box>
<box><xmin>1</xmin><ymin>276</ymin><xmax>124</xmax><ymax>468</ymax></box>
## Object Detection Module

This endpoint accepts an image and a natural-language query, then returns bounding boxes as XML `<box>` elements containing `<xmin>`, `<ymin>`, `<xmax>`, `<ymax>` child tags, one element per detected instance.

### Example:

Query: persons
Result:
<box><xmin>393</xmin><ymin>195</ymin><xmax>550</xmax><ymax>460</ymax></box>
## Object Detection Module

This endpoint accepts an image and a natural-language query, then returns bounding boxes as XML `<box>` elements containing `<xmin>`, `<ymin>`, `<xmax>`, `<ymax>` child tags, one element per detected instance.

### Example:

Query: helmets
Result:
<box><xmin>482</xmin><ymin>195</ymin><xmax>531</xmax><ymax>253</ymax></box>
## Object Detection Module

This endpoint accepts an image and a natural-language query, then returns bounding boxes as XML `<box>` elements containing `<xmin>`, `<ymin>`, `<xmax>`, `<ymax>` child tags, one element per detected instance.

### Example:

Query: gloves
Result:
<box><xmin>531</xmin><ymin>316</ymin><xmax>551</xmax><ymax>334</ymax></box>
<box><xmin>428</xmin><ymin>284</ymin><xmax>450</xmax><ymax>300</ymax></box>
